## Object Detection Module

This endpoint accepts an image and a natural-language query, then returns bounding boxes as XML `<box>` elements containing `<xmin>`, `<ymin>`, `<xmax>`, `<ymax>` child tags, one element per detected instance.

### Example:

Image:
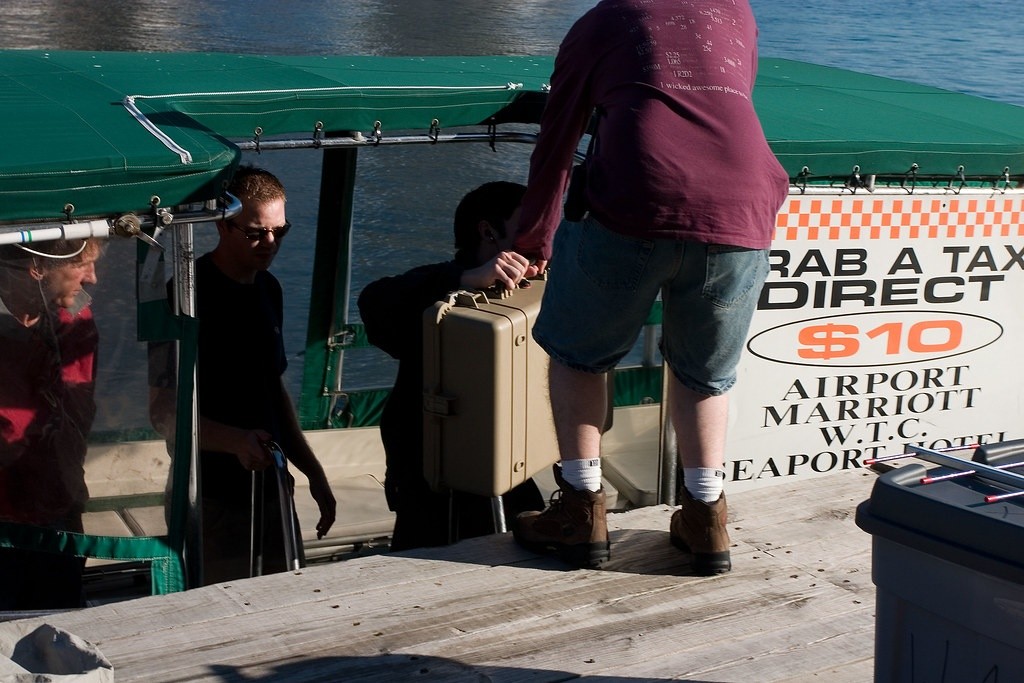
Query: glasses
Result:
<box><xmin>226</xmin><ymin>217</ymin><xmax>291</xmax><ymax>240</ymax></box>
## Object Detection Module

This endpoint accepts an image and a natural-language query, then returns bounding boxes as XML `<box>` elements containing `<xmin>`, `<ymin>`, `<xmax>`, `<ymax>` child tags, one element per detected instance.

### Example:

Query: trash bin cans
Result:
<box><xmin>853</xmin><ymin>439</ymin><xmax>1024</xmax><ymax>683</ymax></box>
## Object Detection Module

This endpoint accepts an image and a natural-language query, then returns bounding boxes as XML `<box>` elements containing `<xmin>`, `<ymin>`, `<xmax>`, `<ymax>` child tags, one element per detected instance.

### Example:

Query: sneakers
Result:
<box><xmin>514</xmin><ymin>463</ymin><xmax>611</xmax><ymax>567</ymax></box>
<box><xmin>669</xmin><ymin>482</ymin><xmax>731</xmax><ymax>573</ymax></box>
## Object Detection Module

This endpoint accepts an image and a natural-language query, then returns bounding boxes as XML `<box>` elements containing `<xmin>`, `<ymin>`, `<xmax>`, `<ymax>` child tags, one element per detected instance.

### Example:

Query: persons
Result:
<box><xmin>149</xmin><ymin>168</ymin><xmax>336</xmax><ymax>586</ymax></box>
<box><xmin>358</xmin><ymin>180</ymin><xmax>546</xmax><ymax>552</ymax></box>
<box><xmin>506</xmin><ymin>1</ymin><xmax>790</xmax><ymax>575</ymax></box>
<box><xmin>0</xmin><ymin>235</ymin><xmax>109</xmax><ymax>608</ymax></box>
